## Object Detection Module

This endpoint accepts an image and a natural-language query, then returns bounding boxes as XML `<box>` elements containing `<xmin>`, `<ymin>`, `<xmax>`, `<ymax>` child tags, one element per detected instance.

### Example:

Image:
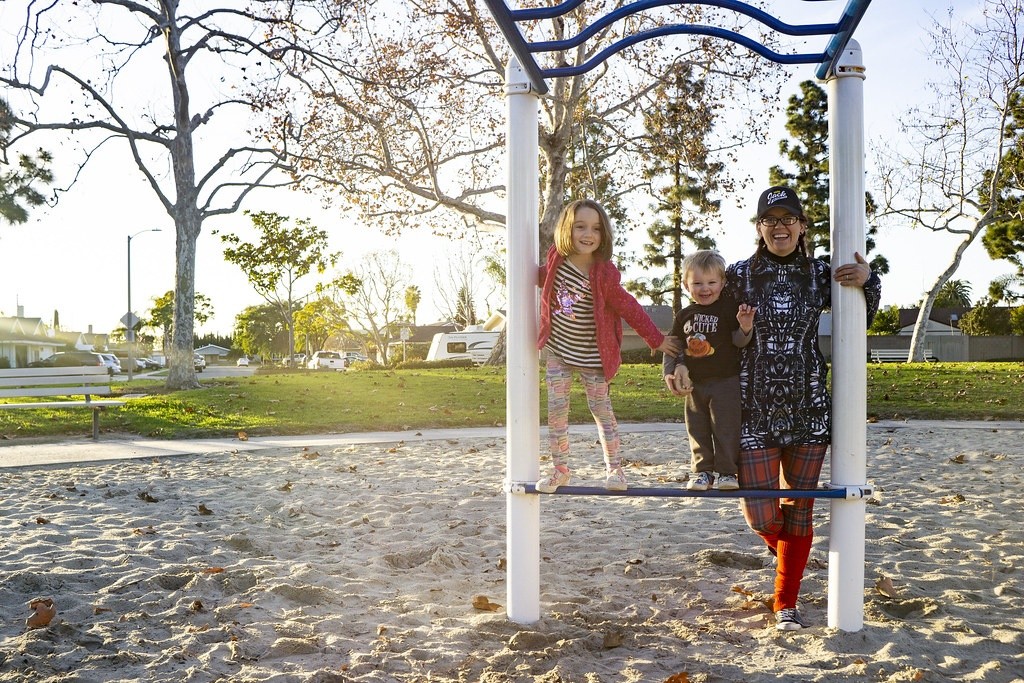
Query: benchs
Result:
<box><xmin>870</xmin><ymin>348</ymin><xmax>933</xmax><ymax>365</ymax></box>
<box><xmin>0</xmin><ymin>364</ymin><xmax>126</xmax><ymax>443</ymax></box>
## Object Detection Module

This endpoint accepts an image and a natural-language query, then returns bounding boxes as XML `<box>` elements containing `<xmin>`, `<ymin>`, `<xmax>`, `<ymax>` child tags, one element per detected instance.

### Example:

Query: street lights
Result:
<box><xmin>127</xmin><ymin>229</ymin><xmax>163</xmax><ymax>381</ymax></box>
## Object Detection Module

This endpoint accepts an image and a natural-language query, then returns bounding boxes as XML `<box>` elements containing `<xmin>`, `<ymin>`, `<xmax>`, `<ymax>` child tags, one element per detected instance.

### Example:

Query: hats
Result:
<box><xmin>756</xmin><ymin>186</ymin><xmax>802</xmax><ymax>219</ymax></box>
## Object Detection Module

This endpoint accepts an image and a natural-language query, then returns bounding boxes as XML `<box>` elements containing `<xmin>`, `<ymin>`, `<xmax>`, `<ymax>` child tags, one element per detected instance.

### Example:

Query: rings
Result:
<box><xmin>847</xmin><ymin>275</ymin><xmax>848</xmax><ymax>280</ymax></box>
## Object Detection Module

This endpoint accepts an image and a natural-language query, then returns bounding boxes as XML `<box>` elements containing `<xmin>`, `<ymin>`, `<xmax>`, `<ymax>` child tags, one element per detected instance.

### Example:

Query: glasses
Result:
<box><xmin>758</xmin><ymin>215</ymin><xmax>800</xmax><ymax>227</ymax></box>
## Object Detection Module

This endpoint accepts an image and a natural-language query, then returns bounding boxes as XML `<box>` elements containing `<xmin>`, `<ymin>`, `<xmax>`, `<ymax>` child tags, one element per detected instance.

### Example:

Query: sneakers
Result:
<box><xmin>535</xmin><ymin>468</ymin><xmax>570</xmax><ymax>493</ymax></box>
<box><xmin>606</xmin><ymin>470</ymin><xmax>628</xmax><ymax>491</ymax></box>
<box><xmin>686</xmin><ymin>472</ymin><xmax>716</xmax><ymax>490</ymax></box>
<box><xmin>772</xmin><ymin>555</ymin><xmax>778</xmax><ymax>568</ymax></box>
<box><xmin>775</xmin><ymin>608</ymin><xmax>812</xmax><ymax>631</ymax></box>
<box><xmin>718</xmin><ymin>474</ymin><xmax>739</xmax><ymax>490</ymax></box>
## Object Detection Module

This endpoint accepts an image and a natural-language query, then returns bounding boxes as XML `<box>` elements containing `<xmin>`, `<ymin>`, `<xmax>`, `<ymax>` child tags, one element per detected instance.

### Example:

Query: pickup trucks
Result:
<box><xmin>307</xmin><ymin>350</ymin><xmax>345</xmax><ymax>371</ymax></box>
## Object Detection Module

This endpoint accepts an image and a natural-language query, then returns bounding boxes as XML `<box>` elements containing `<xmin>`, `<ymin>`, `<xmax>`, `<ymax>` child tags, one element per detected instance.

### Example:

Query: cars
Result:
<box><xmin>136</xmin><ymin>357</ymin><xmax>162</xmax><ymax>371</ymax></box>
<box><xmin>344</xmin><ymin>351</ymin><xmax>367</xmax><ymax>364</ymax></box>
<box><xmin>236</xmin><ymin>358</ymin><xmax>249</xmax><ymax>366</ymax></box>
<box><xmin>195</xmin><ymin>353</ymin><xmax>206</xmax><ymax>373</ymax></box>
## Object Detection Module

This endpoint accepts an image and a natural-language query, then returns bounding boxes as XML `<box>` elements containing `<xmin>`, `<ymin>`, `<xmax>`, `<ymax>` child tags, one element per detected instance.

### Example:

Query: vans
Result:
<box><xmin>283</xmin><ymin>354</ymin><xmax>306</xmax><ymax>366</ymax></box>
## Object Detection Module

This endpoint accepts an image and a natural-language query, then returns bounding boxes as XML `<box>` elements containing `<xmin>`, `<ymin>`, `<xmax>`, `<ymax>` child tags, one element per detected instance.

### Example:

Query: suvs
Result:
<box><xmin>26</xmin><ymin>350</ymin><xmax>121</xmax><ymax>377</ymax></box>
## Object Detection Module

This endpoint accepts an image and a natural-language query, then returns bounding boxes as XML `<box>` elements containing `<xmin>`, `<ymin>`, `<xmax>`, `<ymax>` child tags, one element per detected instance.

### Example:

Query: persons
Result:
<box><xmin>662</xmin><ymin>251</ymin><xmax>756</xmax><ymax>491</ymax></box>
<box><xmin>535</xmin><ymin>200</ymin><xmax>678</xmax><ymax>494</ymax></box>
<box><xmin>673</xmin><ymin>186</ymin><xmax>882</xmax><ymax>631</ymax></box>
